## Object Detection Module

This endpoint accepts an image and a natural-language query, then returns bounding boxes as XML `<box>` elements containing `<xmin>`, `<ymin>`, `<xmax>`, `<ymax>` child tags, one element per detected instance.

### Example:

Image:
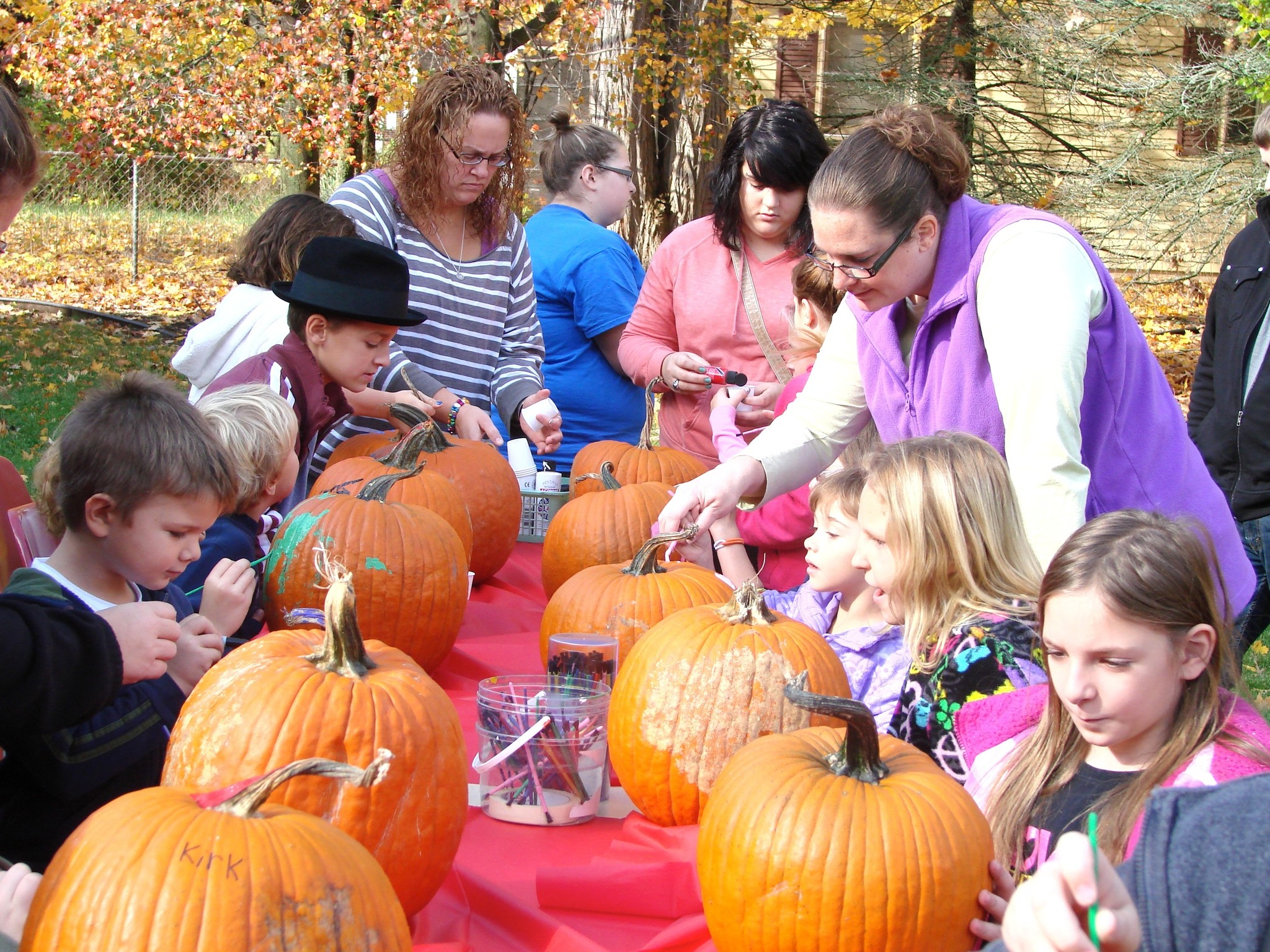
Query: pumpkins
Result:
<box><xmin>540</xmin><ymin>462</ymin><xmax>687</xmax><ymax>603</ymax></box>
<box><xmin>309</xmin><ymin>421</ymin><xmax>474</xmax><ymax>569</ymax></box>
<box><xmin>368</xmin><ymin>403</ymin><xmax>522</xmax><ymax>587</ymax></box>
<box><xmin>16</xmin><ymin>749</ymin><xmax>415</xmax><ymax>951</ymax></box>
<box><xmin>695</xmin><ymin>671</ymin><xmax>997</xmax><ymax>951</ymax></box>
<box><xmin>262</xmin><ymin>460</ymin><xmax>469</xmax><ymax>675</ymax></box>
<box><xmin>567</xmin><ymin>376</ymin><xmax>709</xmax><ymax>499</ymax></box>
<box><xmin>607</xmin><ymin>581</ymin><xmax>852</xmax><ymax>828</ymax></box>
<box><xmin>539</xmin><ymin>524</ymin><xmax>735</xmax><ymax>679</ymax></box>
<box><xmin>157</xmin><ymin>570</ymin><xmax>470</xmax><ymax>920</ymax></box>
<box><xmin>325</xmin><ymin>427</ymin><xmax>456</xmax><ymax>469</ymax></box>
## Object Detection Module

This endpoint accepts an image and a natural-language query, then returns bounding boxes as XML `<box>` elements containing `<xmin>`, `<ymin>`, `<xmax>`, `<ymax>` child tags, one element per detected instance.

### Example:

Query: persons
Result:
<box><xmin>198</xmin><ymin>238</ymin><xmax>428</xmax><ymax>524</ymax></box>
<box><xmin>658</xmin><ymin>106</ymin><xmax>1257</xmax><ymax>631</ymax></box>
<box><xmin>674</xmin><ymin>467</ymin><xmax>912</xmax><ymax>733</ymax></box>
<box><xmin>1187</xmin><ymin>103</ymin><xmax>1270</xmax><ymax>690</ymax></box>
<box><xmin>172</xmin><ymin>192</ymin><xmax>443</xmax><ymax>435</ymax></box>
<box><xmin>954</xmin><ymin>511</ymin><xmax>1270</xmax><ymax>944</ymax></box>
<box><xmin>853</xmin><ymin>429</ymin><xmax>1050</xmax><ymax>808</ymax></box>
<box><xmin>993</xmin><ymin>771</ymin><xmax>1270</xmax><ymax>951</ymax></box>
<box><xmin>708</xmin><ymin>252</ymin><xmax>845</xmax><ymax>593</ymax></box>
<box><xmin>0</xmin><ymin>81</ymin><xmax>179</xmax><ymax>750</ymax></box>
<box><xmin>1</xmin><ymin>369</ymin><xmax>299</xmax><ymax>872</ymax></box>
<box><xmin>618</xmin><ymin>100</ymin><xmax>832</xmax><ymax>457</ymax></box>
<box><xmin>327</xmin><ymin>64</ymin><xmax>563</xmax><ymax>456</ymax></box>
<box><xmin>487</xmin><ymin>108</ymin><xmax>654</xmax><ymax>481</ymax></box>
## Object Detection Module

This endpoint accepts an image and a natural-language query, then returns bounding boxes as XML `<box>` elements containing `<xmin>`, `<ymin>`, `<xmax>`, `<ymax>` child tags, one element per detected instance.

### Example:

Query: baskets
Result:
<box><xmin>516</xmin><ymin>477</ymin><xmax>569</xmax><ymax>543</ymax></box>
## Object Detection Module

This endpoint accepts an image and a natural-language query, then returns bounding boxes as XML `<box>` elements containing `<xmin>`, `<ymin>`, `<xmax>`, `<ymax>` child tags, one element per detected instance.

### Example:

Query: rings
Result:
<box><xmin>673</xmin><ymin>379</ymin><xmax>679</xmax><ymax>390</ymax></box>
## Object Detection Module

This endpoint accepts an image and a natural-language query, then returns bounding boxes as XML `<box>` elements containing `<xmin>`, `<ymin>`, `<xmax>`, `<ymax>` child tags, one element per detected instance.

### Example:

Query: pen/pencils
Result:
<box><xmin>222</xmin><ymin>638</ymin><xmax>248</xmax><ymax>645</ymax></box>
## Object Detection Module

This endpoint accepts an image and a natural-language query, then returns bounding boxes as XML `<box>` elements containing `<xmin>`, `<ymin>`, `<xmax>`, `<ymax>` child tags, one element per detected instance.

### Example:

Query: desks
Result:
<box><xmin>253</xmin><ymin>536</ymin><xmax>724</xmax><ymax>952</ymax></box>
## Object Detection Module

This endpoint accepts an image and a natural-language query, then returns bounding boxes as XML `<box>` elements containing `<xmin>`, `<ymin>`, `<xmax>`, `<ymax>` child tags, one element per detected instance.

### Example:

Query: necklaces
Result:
<box><xmin>430</xmin><ymin>204</ymin><xmax>467</xmax><ymax>281</ymax></box>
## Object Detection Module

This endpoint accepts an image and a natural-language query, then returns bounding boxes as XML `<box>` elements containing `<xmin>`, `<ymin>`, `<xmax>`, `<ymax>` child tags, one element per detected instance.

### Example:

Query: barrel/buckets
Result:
<box><xmin>471</xmin><ymin>674</ymin><xmax>611</xmax><ymax>828</ymax></box>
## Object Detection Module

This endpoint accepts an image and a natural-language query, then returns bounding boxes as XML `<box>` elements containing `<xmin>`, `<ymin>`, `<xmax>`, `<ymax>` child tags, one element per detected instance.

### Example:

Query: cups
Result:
<box><xmin>521</xmin><ymin>397</ymin><xmax>559</xmax><ymax>432</ymax></box>
<box><xmin>506</xmin><ymin>438</ymin><xmax>537</xmax><ymax>478</ymax></box>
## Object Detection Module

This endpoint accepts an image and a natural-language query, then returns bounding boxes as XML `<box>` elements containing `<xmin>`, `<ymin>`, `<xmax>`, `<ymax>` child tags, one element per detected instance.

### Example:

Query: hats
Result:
<box><xmin>270</xmin><ymin>236</ymin><xmax>427</xmax><ymax>326</ymax></box>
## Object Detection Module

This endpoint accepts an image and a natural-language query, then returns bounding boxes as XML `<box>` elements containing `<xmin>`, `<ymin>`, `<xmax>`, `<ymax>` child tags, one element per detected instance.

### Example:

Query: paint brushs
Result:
<box><xmin>184</xmin><ymin>550</ymin><xmax>272</xmax><ymax>597</ymax></box>
<box><xmin>736</xmin><ymin>426</ymin><xmax>767</xmax><ymax>437</ymax></box>
<box><xmin>476</xmin><ymin>682</ymin><xmax>604</xmax><ymax>823</ymax></box>
<box><xmin>547</xmin><ymin>651</ymin><xmax>613</xmax><ymax>694</ymax></box>
<box><xmin>1086</xmin><ymin>812</ymin><xmax>1098</xmax><ymax>949</ymax></box>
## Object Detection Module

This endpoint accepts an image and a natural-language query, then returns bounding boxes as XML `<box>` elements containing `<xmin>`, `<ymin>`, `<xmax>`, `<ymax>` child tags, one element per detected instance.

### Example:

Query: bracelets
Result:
<box><xmin>713</xmin><ymin>539</ymin><xmax>745</xmax><ymax>550</ymax></box>
<box><xmin>447</xmin><ymin>397</ymin><xmax>469</xmax><ymax>435</ymax></box>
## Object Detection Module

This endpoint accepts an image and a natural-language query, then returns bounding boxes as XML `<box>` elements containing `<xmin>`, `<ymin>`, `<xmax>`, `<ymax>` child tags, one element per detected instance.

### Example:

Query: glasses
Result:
<box><xmin>806</xmin><ymin>218</ymin><xmax>922</xmax><ymax>279</ymax></box>
<box><xmin>580</xmin><ymin>164</ymin><xmax>634</xmax><ymax>184</ymax></box>
<box><xmin>434</xmin><ymin>125</ymin><xmax>511</xmax><ymax>166</ymax></box>
